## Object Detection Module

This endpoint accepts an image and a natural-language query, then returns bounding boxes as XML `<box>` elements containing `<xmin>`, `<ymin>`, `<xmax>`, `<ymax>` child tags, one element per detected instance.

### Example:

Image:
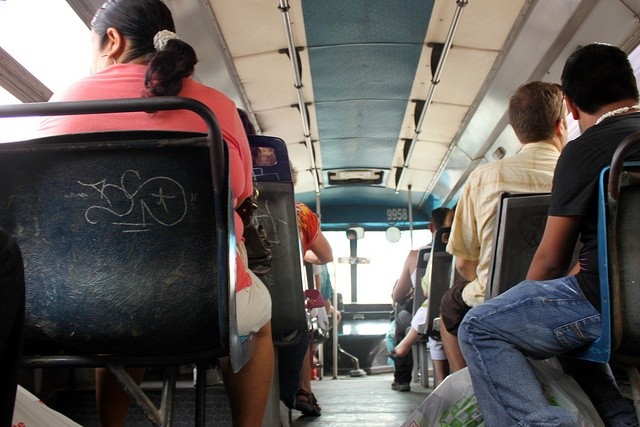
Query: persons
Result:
<box><xmin>259</xmin><ymin>140</ymin><xmax>334</xmax><ymax>416</ymax></box>
<box><xmin>439</xmin><ymin>81</ymin><xmax>570</xmax><ymax>371</ymax></box>
<box><xmin>38</xmin><ymin>0</ymin><xmax>275</xmax><ymax>427</ymax></box>
<box><xmin>386</xmin><ymin>203</ymin><xmax>470</xmax><ymax>387</ymax></box>
<box><xmin>457</xmin><ymin>42</ymin><xmax>640</xmax><ymax>427</ymax></box>
<box><xmin>391</xmin><ymin>208</ymin><xmax>452</xmax><ymax>391</ymax></box>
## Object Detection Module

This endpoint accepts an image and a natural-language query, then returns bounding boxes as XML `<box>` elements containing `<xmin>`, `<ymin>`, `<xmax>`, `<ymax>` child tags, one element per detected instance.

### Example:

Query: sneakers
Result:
<box><xmin>391</xmin><ymin>380</ymin><xmax>411</xmax><ymax>392</ymax></box>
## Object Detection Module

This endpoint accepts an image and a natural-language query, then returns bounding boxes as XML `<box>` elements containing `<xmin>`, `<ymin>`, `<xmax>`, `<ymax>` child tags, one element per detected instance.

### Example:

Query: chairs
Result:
<box><xmin>404</xmin><ymin>247</ymin><xmax>435</xmax><ymax>388</ymax></box>
<box><xmin>236</xmin><ymin>133</ymin><xmax>310</xmax><ymax>426</ymax></box>
<box><xmin>419</xmin><ymin>227</ymin><xmax>466</xmax><ymax>390</ymax></box>
<box><xmin>571</xmin><ymin>129</ymin><xmax>639</xmax><ymax>426</ymax></box>
<box><xmin>304</xmin><ymin>262</ymin><xmax>314</xmax><ymax>291</ymax></box>
<box><xmin>483</xmin><ymin>189</ymin><xmax>581</xmax><ymax>301</ymax></box>
<box><xmin>0</xmin><ymin>94</ymin><xmax>260</xmax><ymax>426</ymax></box>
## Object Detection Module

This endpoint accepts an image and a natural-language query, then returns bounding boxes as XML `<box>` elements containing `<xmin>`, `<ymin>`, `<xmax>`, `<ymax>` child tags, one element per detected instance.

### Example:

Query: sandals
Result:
<box><xmin>291</xmin><ymin>388</ymin><xmax>321</xmax><ymax>416</ymax></box>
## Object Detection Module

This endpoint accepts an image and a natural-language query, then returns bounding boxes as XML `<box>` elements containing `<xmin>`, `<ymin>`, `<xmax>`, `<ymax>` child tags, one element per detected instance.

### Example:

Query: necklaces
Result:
<box><xmin>596</xmin><ymin>104</ymin><xmax>640</xmax><ymax>126</ymax></box>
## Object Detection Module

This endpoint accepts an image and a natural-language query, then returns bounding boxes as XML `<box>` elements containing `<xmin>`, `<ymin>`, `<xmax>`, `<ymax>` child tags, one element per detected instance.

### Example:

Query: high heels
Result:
<box><xmin>386</xmin><ymin>350</ymin><xmax>406</xmax><ymax>366</ymax></box>
